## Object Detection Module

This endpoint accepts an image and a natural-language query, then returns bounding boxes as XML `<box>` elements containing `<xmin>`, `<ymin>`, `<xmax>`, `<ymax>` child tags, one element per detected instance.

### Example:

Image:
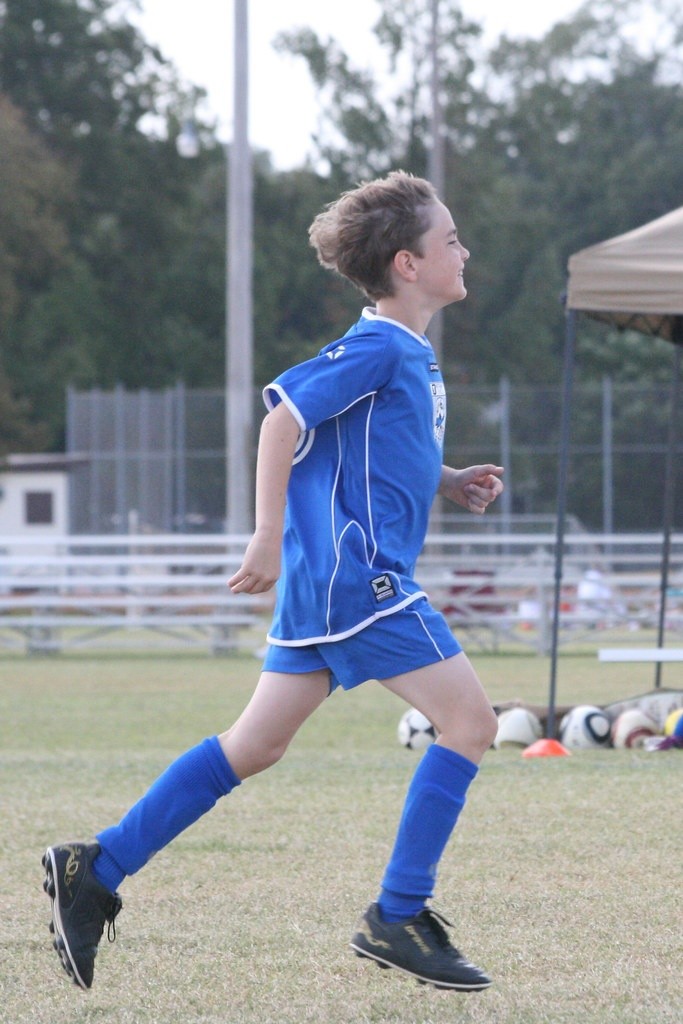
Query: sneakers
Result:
<box><xmin>348</xmin><ymin>902</ymin><xmax>494</xmax><ymax>993</ymax></box>
<box><xmin>40</xmin><ymin>842</ymin><xmax>123</xmax><ymax>991</ymax></box>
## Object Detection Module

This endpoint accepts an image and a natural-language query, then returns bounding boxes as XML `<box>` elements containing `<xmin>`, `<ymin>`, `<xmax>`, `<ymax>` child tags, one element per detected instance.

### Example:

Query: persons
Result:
<box><xmin>36</xmin><ymin>165</ymin><xmax>502</xmax><ymax>992</ymax></box>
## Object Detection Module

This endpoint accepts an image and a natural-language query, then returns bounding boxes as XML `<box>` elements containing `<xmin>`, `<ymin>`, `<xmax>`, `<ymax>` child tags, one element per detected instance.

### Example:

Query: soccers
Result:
<box><xmin>398</xmin><ymin>704</ymin><xmax>682</xmax><ymax>750</ymax></box>
